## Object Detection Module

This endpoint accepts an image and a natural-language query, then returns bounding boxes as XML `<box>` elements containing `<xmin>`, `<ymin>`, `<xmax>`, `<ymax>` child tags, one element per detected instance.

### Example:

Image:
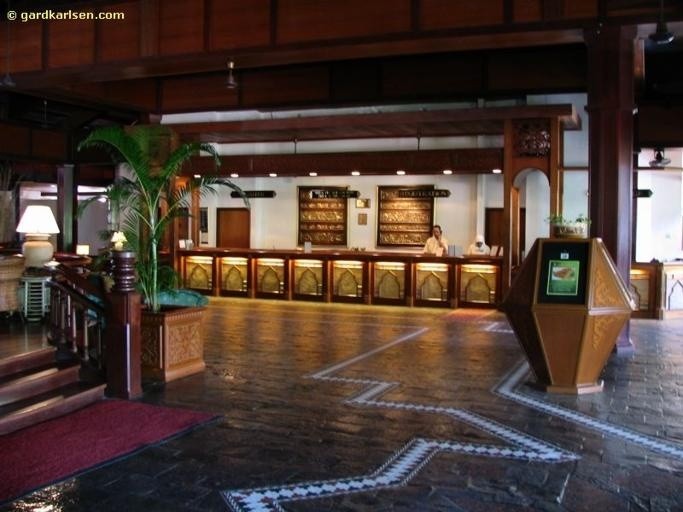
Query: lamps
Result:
<box><xmin>110</xmin><ymin>231</ymin><xmax>128</xmax><ymax>250</ymax></box>
<box><xmin>650</xmin><ymin>149</ymin><xmax>671</xmax><ymax>168</ymax></box>
<box><xmin>15</xmin><ymin>205</ymin><xmax>62</xmax><ymax>269</ymax></box>
<box><xmin>648</xmin><ymin>22</ymin><xmax>675</xmax><ymax>46</ymax></box>
<box><xmin>224</xmin><ymin>70</ymin><xmax>237</xmax><ymax>90</ymax></box>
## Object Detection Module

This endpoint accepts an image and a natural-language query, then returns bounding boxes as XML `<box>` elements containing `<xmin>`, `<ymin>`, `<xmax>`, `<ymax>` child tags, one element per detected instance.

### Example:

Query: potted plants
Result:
<box><xmin>545</xmin><ymin>213</ymin><xmax>593</xmax><ymax>238</ymax></box>
<box><xmin>73</xmin><ymin>128</ymin><xmax>251</xmax><ymax>385</ymax></box>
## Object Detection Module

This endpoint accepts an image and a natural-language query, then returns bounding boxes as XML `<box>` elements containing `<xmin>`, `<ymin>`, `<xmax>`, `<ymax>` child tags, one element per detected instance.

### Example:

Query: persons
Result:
<box><xmin>424</xmin><ymin>224</ymin><xmax>448</xmax><ymax>256</ymax></box>
<box><xmin>468</xmin><ymin>236</ymin><xmax>489</xmax><ymax>254</ymax></box>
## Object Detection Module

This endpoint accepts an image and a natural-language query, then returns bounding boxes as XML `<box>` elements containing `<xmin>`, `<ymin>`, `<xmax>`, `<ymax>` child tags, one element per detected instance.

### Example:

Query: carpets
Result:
<box><xmin>0</xmin><ymin>398</ymin><xmax>225</xmax><ymax>507</ymax></box>
